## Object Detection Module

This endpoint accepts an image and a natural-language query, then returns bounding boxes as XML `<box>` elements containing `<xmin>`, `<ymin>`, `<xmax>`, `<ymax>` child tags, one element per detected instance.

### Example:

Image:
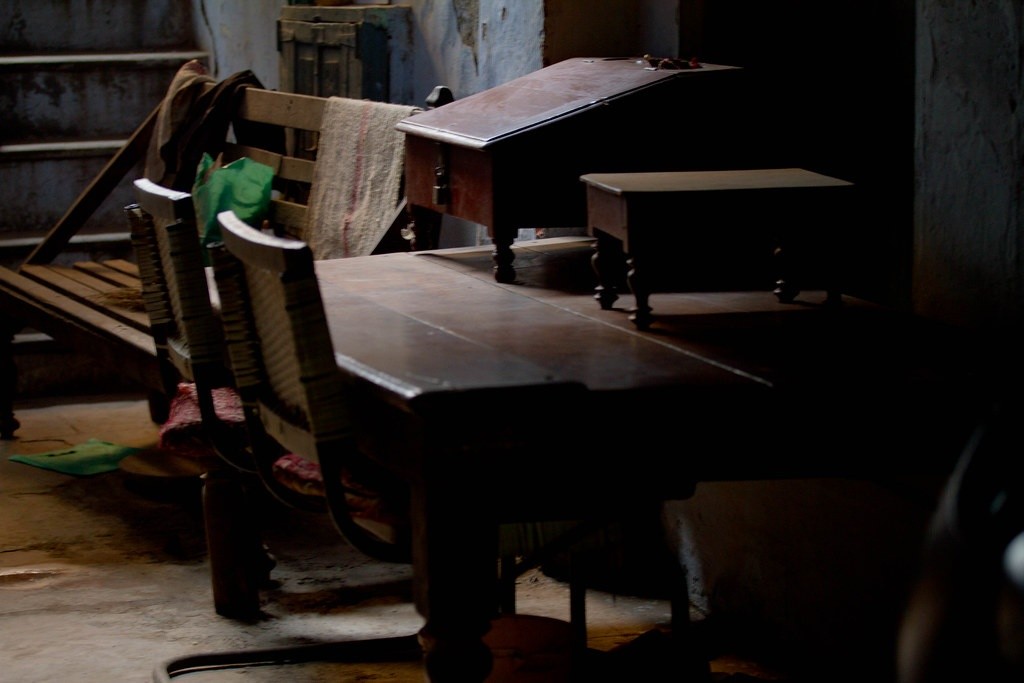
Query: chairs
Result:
<box><xmin>123</xmin><ymin>177</ymin><xmax>608</xmax><ymax>672</ymax></box>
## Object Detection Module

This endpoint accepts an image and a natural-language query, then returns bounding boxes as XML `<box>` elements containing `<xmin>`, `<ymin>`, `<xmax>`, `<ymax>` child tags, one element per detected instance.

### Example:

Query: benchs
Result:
<box><xmin>0</xmin><ymin>56</ymin><xmax>455</xmax><ymax>440</ymax></box>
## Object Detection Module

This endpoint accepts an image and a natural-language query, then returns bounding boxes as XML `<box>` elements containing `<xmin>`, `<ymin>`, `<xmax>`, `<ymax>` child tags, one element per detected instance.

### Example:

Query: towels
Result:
<box><xmin>301</xmin><ymin>95</ymin><xmax>423</xmax><ymax>259</ymax></box>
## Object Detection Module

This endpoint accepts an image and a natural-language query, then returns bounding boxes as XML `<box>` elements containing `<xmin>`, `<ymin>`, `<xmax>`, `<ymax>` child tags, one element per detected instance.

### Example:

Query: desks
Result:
<box><xmin>579</xmin><ymin>167</ymin><xmax>854</xmax><ymax>327</ymax></box>
<box><xmin>204</xmin><ymin>236</ymin><xmax>899</xmax><ymax>683</ymax></box>
